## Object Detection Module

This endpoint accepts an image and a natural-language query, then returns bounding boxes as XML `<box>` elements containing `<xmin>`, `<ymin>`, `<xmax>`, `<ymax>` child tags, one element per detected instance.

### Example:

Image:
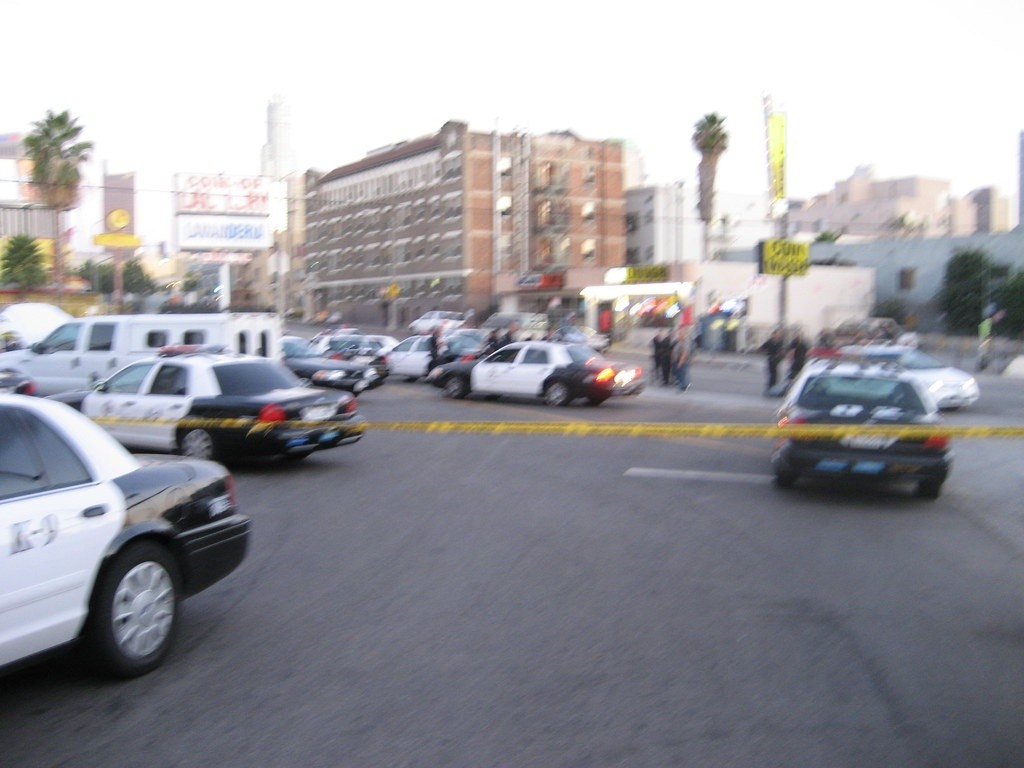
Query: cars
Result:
<box><xmin>429</xmin><ymin>340</ymin><xmax>647</xmax><ymax>409</ymax></box>
<box><xmin>766</xmin><ymin>343</ymin><xmax>954</xmax><ymax>501</ymax></box>
<box><xmin>0</xmin><ymin>391</ymin><xmax>256</xmax><ymax>683</ymax></box>
<box><xmin>835</xmin><ymin>345</ymin><xmax>982</xmax><ymax>411</ymax></box>
<box><xmin>279</xmin><ymin>336</ymin><xmax>382</xmax><ymax>401</ymax></box>
<box><xmin>37</xmin><ymin>342</ymin><xmax>369</xmax><ymax>473</ymax></box>
<box><xmin>288</xmin><ymin>309</ymin><xmax>612</xmax><ymax>376</ymax></box>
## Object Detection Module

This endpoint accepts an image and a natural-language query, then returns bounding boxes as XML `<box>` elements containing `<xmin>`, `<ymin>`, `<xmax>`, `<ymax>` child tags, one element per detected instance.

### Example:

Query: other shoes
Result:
<box><xmin>682</xmin><ymin>383</ymin><xmax>692</xmax><ymax>394</ymax></box>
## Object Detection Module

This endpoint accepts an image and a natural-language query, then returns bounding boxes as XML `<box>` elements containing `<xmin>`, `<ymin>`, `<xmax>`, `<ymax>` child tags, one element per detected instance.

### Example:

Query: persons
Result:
<box><xmin>430</xmin><ymin>321</ymin><xmax>811</xmax><ymax>395</ymax></box>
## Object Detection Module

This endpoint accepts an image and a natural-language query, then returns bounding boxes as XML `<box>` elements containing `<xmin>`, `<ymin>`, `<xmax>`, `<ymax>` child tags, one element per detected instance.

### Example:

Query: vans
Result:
<box><xmin>0</xmin><ymin>311</ymin><xmax>283</xmax><ymax>409</ymax></box>
